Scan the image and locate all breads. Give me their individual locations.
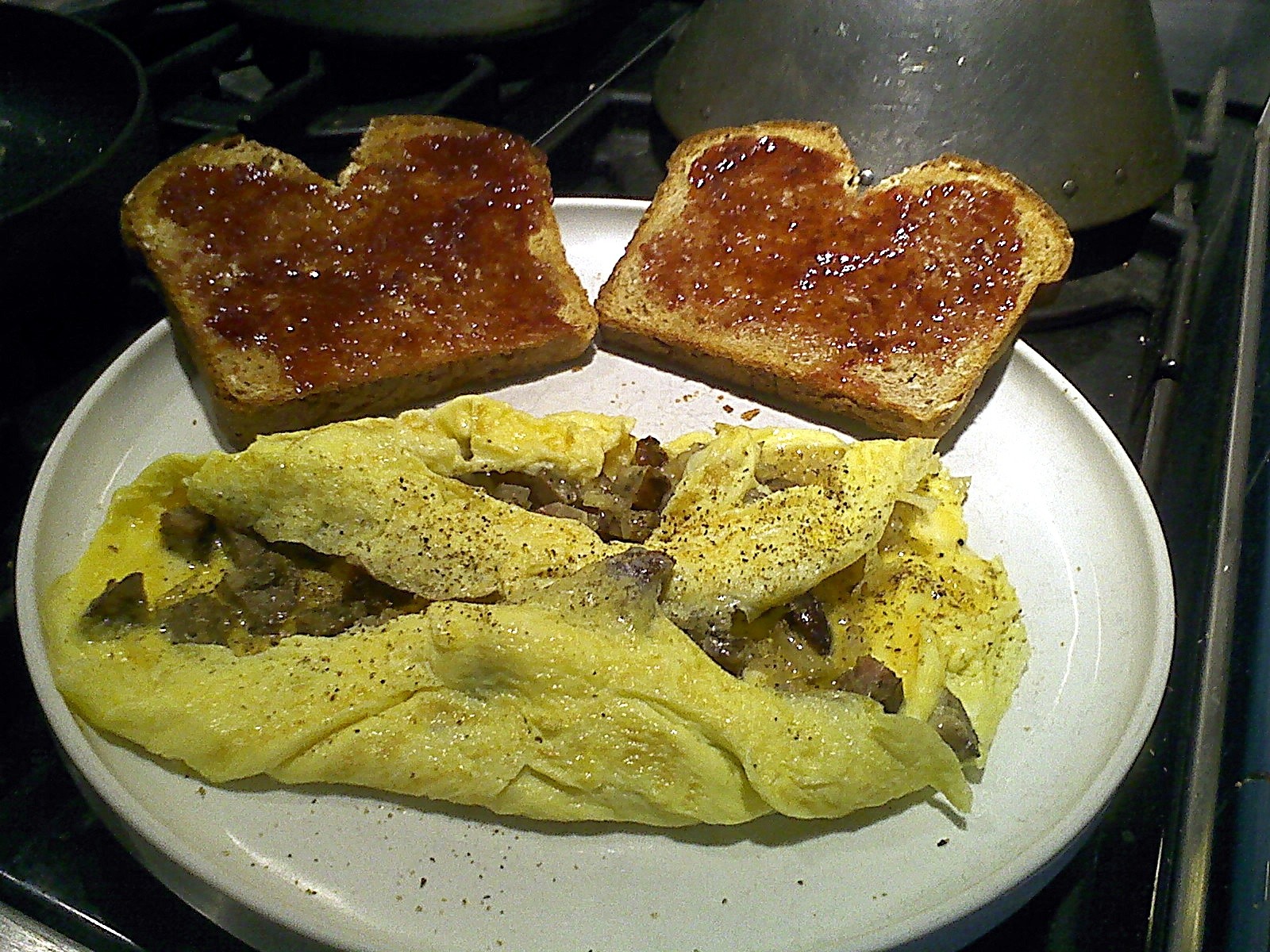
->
[121,115,598,454]
[593,120,1074,439]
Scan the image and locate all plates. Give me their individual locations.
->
[15,194,1179,952]
[1,1,149,219]
